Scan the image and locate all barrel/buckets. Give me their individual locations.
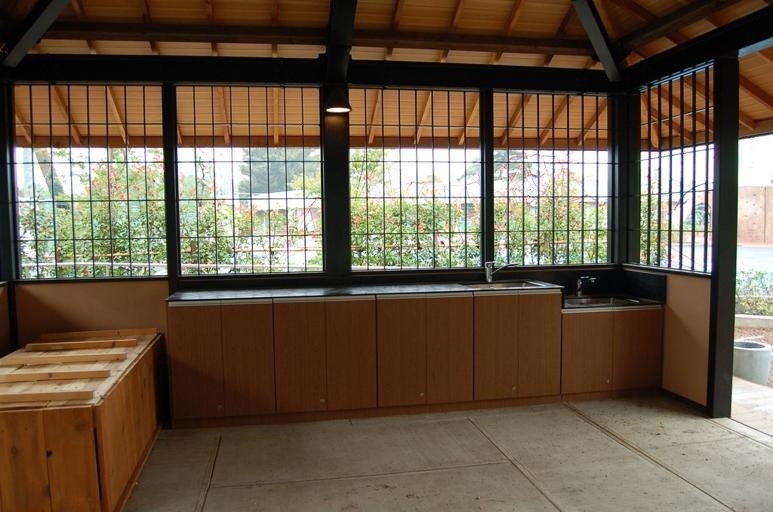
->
[732,338,773,384]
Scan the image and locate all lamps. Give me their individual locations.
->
[321,38,352,114]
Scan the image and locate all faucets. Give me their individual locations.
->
[492,262,520,277]
[576,274,597,298]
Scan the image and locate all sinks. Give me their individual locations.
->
[460,279,542,289]
[565,296,633,307]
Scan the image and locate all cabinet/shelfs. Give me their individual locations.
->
[0,332,162,510]
